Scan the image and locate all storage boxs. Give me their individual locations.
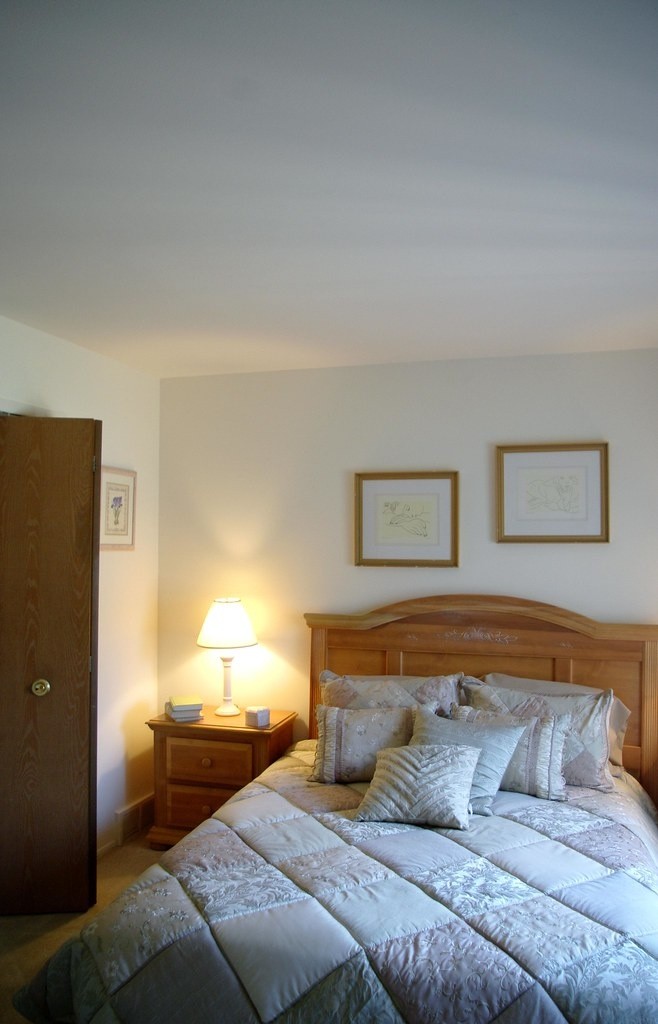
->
[244,706,270,727]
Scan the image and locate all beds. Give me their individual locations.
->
[14,594,658,1024]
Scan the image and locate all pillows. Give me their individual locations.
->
[306,669,633,831]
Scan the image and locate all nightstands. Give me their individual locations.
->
[144,705,298,850]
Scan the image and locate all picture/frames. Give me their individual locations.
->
[496,441,611,544]
[100,465,138,551]
[353,470,459,568]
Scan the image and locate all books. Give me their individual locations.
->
[165,695,205,722]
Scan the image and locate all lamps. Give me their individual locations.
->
[196,598,257,716]
